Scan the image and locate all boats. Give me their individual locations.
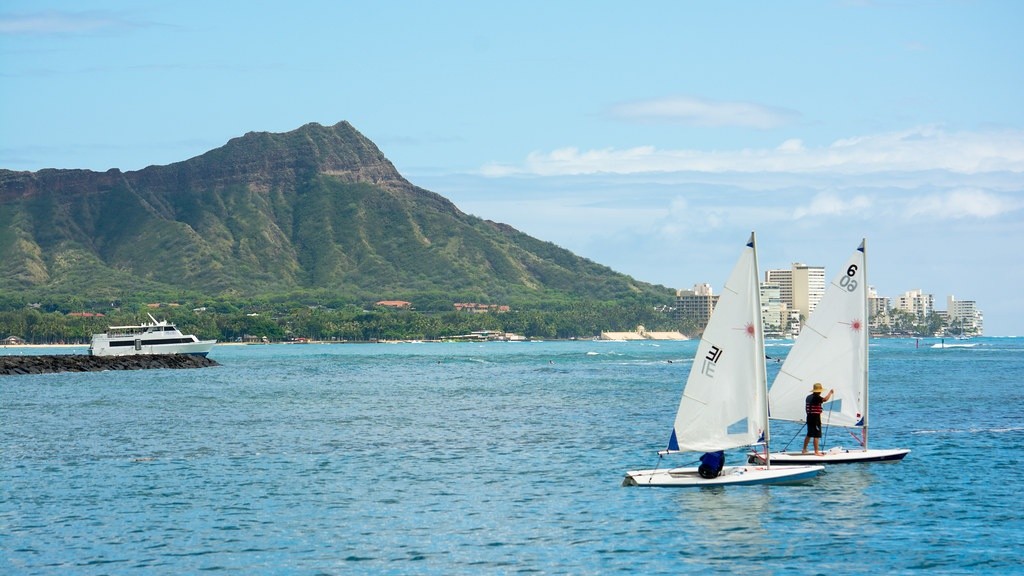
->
[87,311,217,357]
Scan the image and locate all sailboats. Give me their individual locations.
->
[744,237,912,464]
[622,232,826,489]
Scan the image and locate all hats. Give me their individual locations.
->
[809,383,824,392]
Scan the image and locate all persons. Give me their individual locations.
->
[802,384,834,456]
[698,450,725,478]
